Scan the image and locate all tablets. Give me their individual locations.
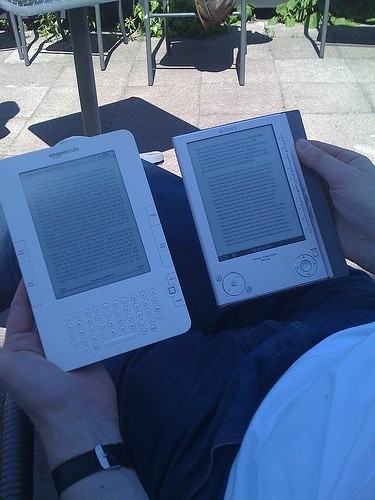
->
[173,112,333,309]
[0,129,191,374]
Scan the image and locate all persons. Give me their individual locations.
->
[0,134,375,500]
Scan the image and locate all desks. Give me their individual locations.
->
[1,0,118,139]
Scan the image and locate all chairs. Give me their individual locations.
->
[10,1,127,71]
[144,1,246,86]
[303,0,331,58]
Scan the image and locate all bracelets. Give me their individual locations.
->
[52,441,136,498]
